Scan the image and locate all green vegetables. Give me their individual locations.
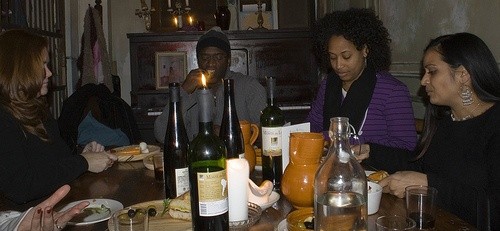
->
[160,199,170,216]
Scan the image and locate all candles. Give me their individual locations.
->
[185,0,189,6]
[167,0,171,8]
[224,157,250,225]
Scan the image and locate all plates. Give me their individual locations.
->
[277,218,288,231]
[108,199,194,231]
[363,169,377,176]
[108,145,160,161]
[59,198,124,225]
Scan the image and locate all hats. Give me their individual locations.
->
[195,25,231,55]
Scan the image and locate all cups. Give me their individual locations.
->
[113,207,149,231]
[405,185,439,231]
[375,215,416,231]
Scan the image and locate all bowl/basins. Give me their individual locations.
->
[348,180,382,215]
[288,209,336,231]
[229,202,261,231]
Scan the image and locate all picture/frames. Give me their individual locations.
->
[155,51,188,91]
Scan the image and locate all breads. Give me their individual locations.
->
[368,170,388,181]
[168,190,192,221]
[118,146,140,155]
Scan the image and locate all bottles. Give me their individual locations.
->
[279,132,329,208]
[164,82,189,199]
[189,87,229,231]
[239,120,259,172]
[219,79,243,159]
[217,6,231,31]
[313,117,369,231]
[260,75,285,189]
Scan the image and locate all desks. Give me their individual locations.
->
[17,158,477,231]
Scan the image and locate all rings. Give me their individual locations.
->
[56,224,64,229]
[108,159,112,165]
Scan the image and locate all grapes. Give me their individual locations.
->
[127,205,156,218]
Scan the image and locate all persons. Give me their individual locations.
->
[351,33,500,231]
[0,184,90,231]
[0,29,114,218]
[153,29,267,143]
[305,7,417,151]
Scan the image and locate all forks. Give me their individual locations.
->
[116,154,134,163]
